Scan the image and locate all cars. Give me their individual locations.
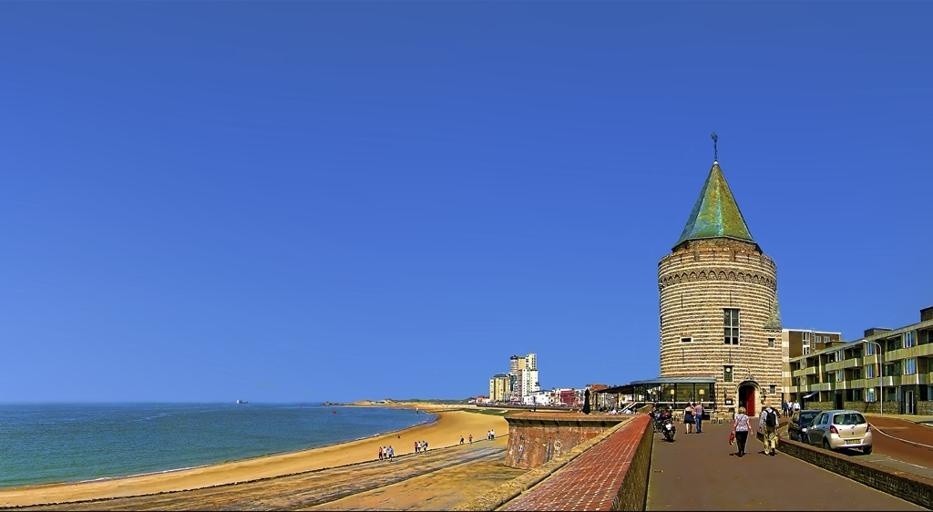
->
[787,409,872,455]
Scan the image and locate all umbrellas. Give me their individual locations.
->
[583,389,590,414]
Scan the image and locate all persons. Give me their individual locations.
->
[683,402,705,434]
[597,403,639,415]
[378,445,395,461]
[757,400,780,456]
[469,434,472,444]
[414,440,428,454]
[781,399,801,418]
[459,435,464,445]
[487,429,495,439]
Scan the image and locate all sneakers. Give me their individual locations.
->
[735,451,744,457]
[766,447,775,456]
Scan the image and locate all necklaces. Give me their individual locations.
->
[732,406,752,458]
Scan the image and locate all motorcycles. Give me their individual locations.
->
[648,409,676,442]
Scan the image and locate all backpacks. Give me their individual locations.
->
[764,409,776,426]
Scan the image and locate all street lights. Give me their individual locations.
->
[861,339,883,416]
